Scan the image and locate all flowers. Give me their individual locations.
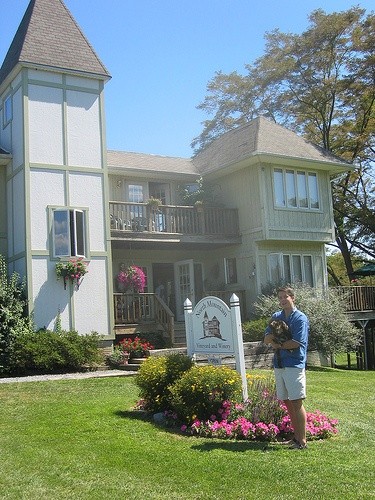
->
[55,256,88,285]
[114,263,147,293]
[118,336,154,352]
[120,350,131,361]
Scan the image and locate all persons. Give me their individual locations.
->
[263,287,308,449]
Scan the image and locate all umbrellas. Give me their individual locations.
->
[351,264,375,276]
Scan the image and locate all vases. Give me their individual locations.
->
[124,360,128,365]
[133,351,143,358]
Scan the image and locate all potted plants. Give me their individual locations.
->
[195,200,204,213]
[146,198,161,210]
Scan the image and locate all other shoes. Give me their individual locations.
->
[290,444,306,449]
[289,439,298,444]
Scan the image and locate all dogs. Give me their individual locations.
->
[269,320,293,369]
